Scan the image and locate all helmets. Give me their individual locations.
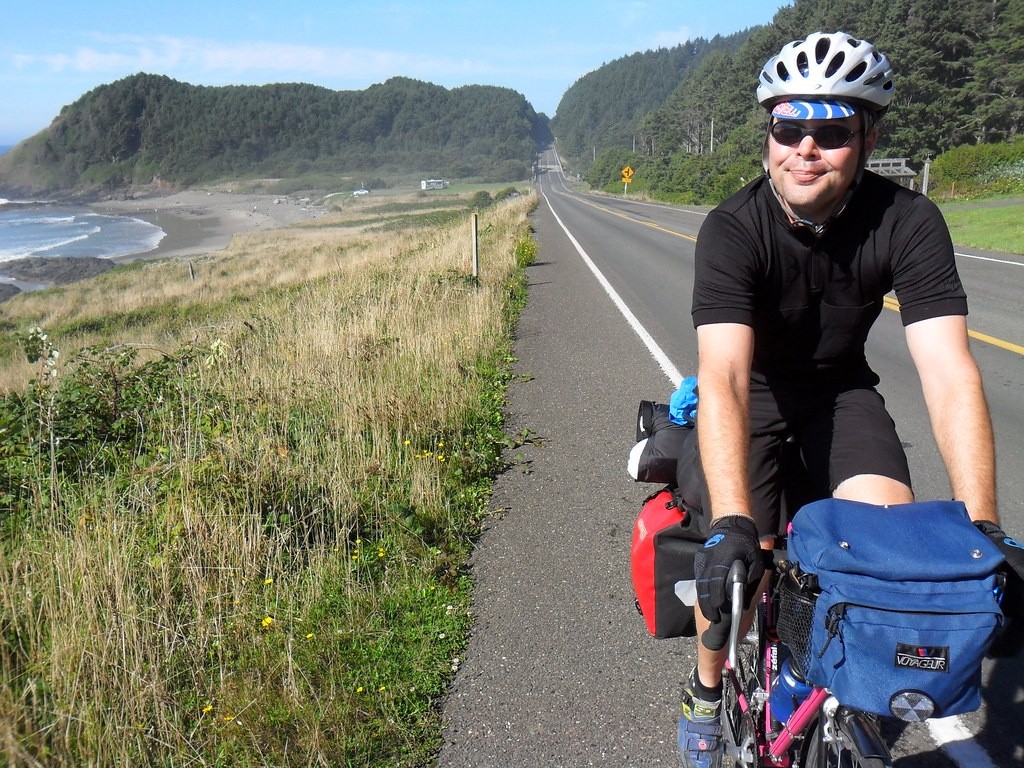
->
[757,29,899,122]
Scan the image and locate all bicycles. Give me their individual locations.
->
[720,432,1017,768]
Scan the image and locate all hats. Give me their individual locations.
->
[772,63,856,121]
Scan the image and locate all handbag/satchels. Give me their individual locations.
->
[629,489,697,638]
[777,498,1006,716]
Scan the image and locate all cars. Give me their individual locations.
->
[352,189,369,196]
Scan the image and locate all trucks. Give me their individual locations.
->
[421,180,450,190]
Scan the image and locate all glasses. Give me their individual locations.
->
[766,114,863,148]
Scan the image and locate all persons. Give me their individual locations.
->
[677,32,1024,768]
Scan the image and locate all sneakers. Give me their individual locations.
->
[677,664,728,768]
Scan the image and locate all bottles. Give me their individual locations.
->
[768,657,813,725]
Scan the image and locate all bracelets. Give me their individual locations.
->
[710,513,756,531]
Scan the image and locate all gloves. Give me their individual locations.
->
[971,520,1024,626]
[699,513,766,620]
